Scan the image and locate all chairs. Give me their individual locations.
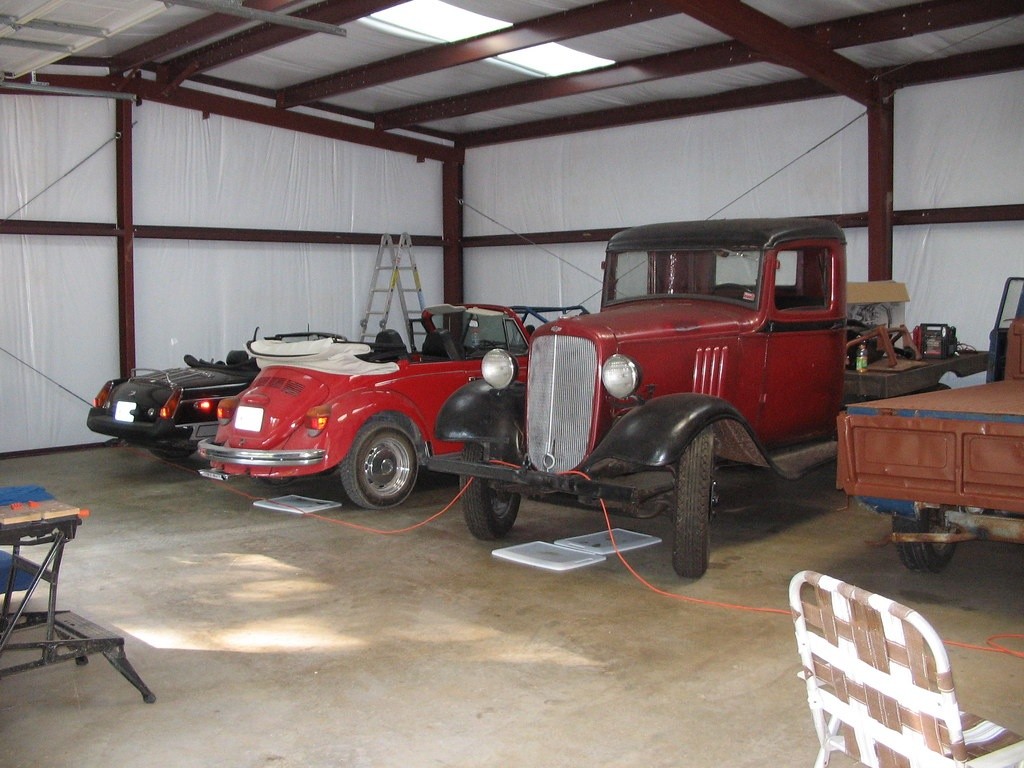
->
[424,329,467,361]
[375,329,409,364]
[789,570,1024,768]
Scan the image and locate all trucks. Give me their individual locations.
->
[425,215,988,582]
[834,275,1024,573]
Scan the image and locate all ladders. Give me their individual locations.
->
[358,232,427,354]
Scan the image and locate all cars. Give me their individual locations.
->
[86,329,347,461]
[198,304,536,511]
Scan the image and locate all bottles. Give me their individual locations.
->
[856,343,868,375]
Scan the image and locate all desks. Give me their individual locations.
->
[0,485,158,704]
